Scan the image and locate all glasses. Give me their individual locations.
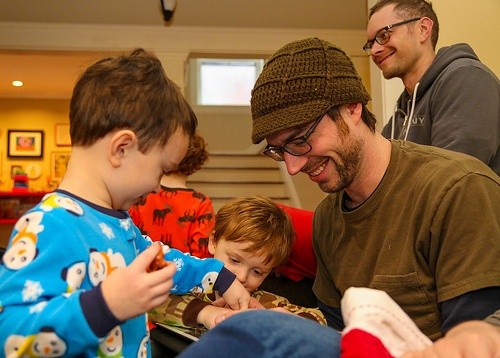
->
[362,17,421,56]
[262,112,325,161]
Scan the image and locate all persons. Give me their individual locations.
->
[363,0,500,177]
[147,36,500,358]
[0,48,251,358]
[127,128,217,330]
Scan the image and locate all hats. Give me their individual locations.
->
[251,36,372,144]
